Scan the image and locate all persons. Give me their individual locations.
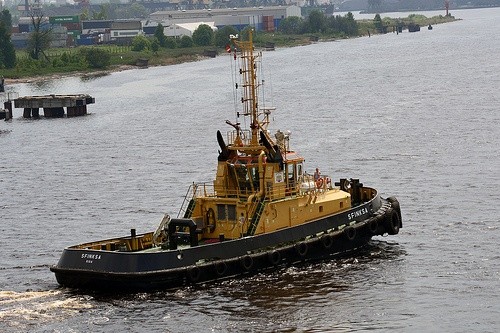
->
[303,170,311,183]
[313,168,322,188]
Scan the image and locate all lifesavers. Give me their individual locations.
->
[317,178,324,188]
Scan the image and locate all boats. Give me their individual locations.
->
[50,27,403,294]
